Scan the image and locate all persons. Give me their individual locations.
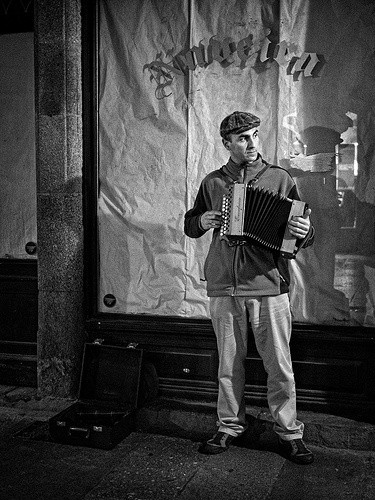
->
[182,112,314,459]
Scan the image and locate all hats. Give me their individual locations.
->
[220,111,260,139]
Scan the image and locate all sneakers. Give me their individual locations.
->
[278,434,314,465]
[198,431,235,454]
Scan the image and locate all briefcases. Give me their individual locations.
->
[47,339,146,450]
